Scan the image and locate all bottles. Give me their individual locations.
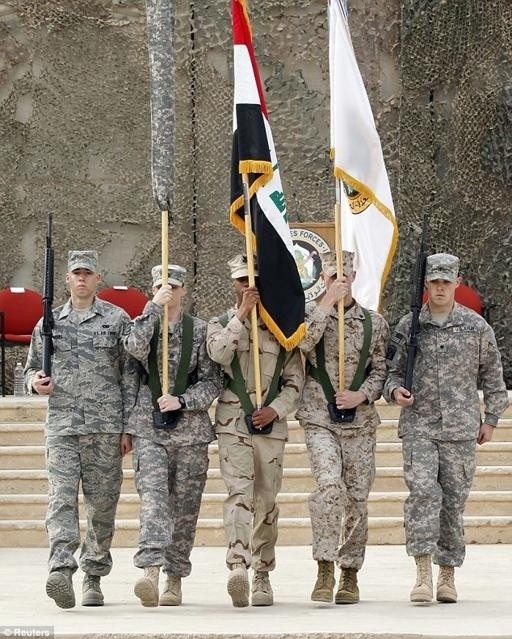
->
[13,362,26,397]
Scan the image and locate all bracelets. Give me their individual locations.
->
[178,395,185,408]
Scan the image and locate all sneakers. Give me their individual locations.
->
[46,567,75,608]
[81,574,104,606]
[311,560,335,602]
[252,570,273,606]
[135,566,160,607]
[411,554,433,601]
[160,576,182,605]
[227,563,249,607]
[436,566,457,602]
[335,568,359,603]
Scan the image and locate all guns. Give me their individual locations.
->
[404,213,427,399]
[41,214,54,386]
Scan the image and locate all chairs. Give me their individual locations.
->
[96,284,148,320]
[0,287,47,344]
[422,284,498,328]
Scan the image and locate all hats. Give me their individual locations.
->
[425,254,460,282]
[320,251,355,277]
[151,265,187,287]
[227,254,260,278]
[68,251,98,273]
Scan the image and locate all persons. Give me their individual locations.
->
[206,253,304,607]
[295,250,388,608]
[24,248,136,610]
[124,264,223,608]
[383,253,510,605]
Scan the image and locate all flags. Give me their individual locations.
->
[227,3,307,351]
[326,2,398,312]
[146,0,175,210]
